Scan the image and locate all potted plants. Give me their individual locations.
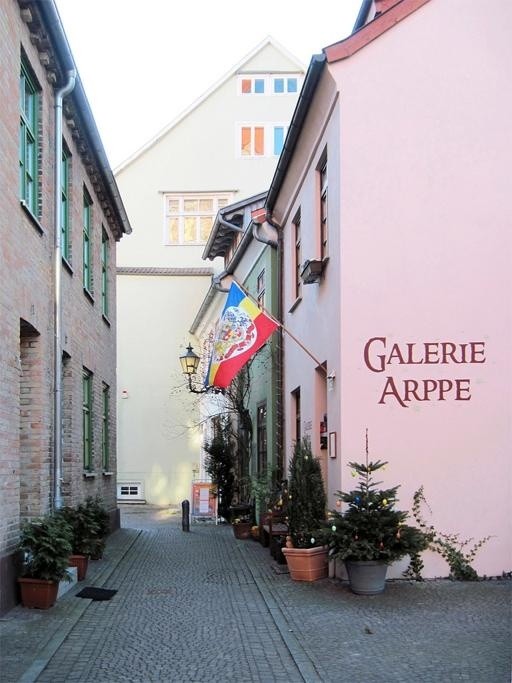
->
[279,435,435,597]
[13,496,112,609]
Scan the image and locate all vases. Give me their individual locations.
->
[234,518,252,538]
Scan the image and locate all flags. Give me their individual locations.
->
[204,279,280,389]
